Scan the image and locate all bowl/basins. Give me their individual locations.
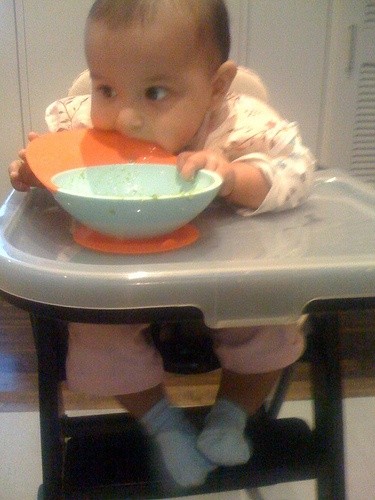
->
[25,128,223,254]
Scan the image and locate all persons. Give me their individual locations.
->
[10,0,316,486]
